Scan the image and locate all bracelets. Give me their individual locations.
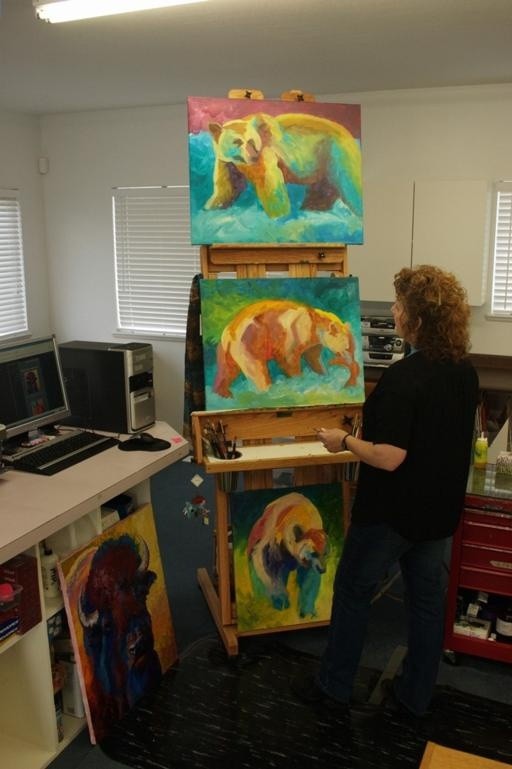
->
[342,433,353,450]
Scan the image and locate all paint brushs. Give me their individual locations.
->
[201,418,239,459]
[340,413,362,482]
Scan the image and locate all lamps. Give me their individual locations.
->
[35,2,192,25]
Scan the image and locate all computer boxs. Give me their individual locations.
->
[55,340,156,435]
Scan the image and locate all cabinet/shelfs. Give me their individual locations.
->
[444,419,511,663]
[191,89,361,656]
[363,180,492,306]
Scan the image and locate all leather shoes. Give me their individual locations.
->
[290,675,351,715]
[380,678,432,724]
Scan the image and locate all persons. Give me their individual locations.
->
[286,263,480,719]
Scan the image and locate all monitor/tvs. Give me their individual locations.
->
[0,334,72,464]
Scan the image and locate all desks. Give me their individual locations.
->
[0,420,192,769]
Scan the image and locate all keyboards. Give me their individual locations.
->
[13,430,121,477]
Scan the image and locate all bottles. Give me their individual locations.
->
[42,550,59,598]
[474,437,488,463]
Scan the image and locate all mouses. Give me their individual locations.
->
[127,433,154,445]
